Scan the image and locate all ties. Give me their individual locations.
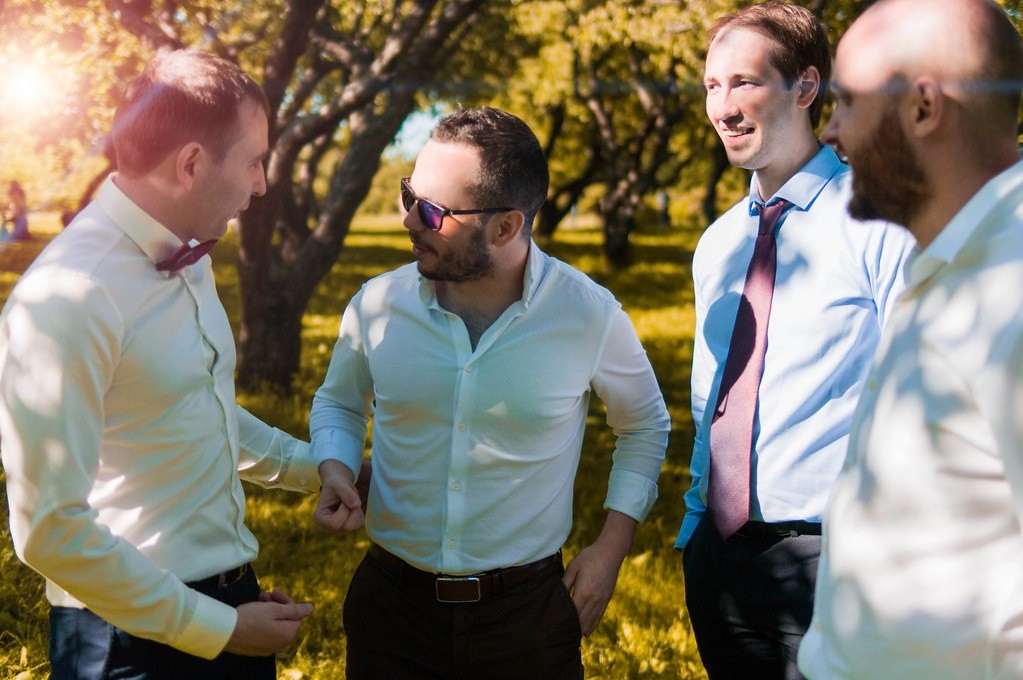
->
[707,199,792,539]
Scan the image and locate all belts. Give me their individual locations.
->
[703,514,821,537]
[368,542,559,603]
[183,561,249,592]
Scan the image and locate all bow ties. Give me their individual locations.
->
[154,238,218,273]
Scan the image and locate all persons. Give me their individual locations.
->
[0,46,371,680]
[799,0,1023,679]
[308,108,671,680]
[672,0,914,680]
[0,180,29,241]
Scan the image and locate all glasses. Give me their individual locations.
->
[400,176,520,233]
